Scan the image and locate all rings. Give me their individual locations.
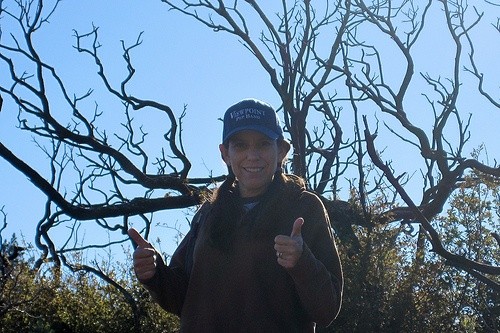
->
[276,251,284,256]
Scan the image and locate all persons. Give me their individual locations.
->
[127,98,338,333]
